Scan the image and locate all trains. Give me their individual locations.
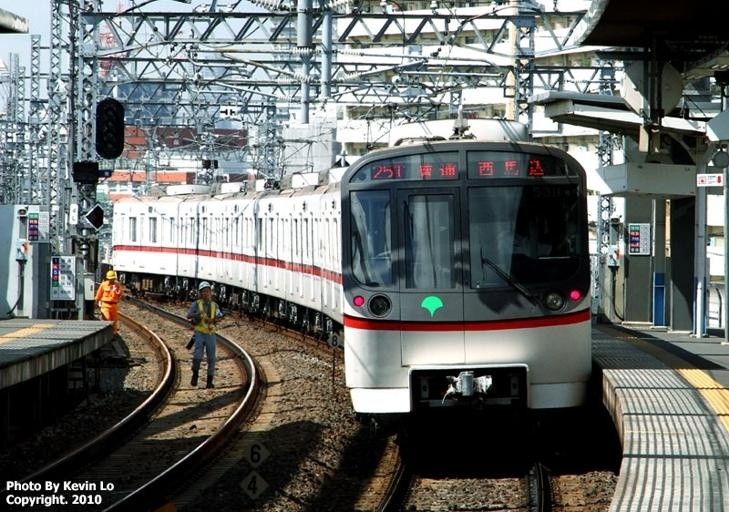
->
[110,138,593,455]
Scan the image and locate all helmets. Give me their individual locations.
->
[106,270,117,279]
[198,281,211,291]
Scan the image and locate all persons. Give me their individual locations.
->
[94,270,123,341]
[186,280,223,388]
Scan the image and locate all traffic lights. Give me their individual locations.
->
[97,97,125,159]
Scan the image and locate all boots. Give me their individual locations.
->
[207,375,214,388]
[191,371,198,386]
